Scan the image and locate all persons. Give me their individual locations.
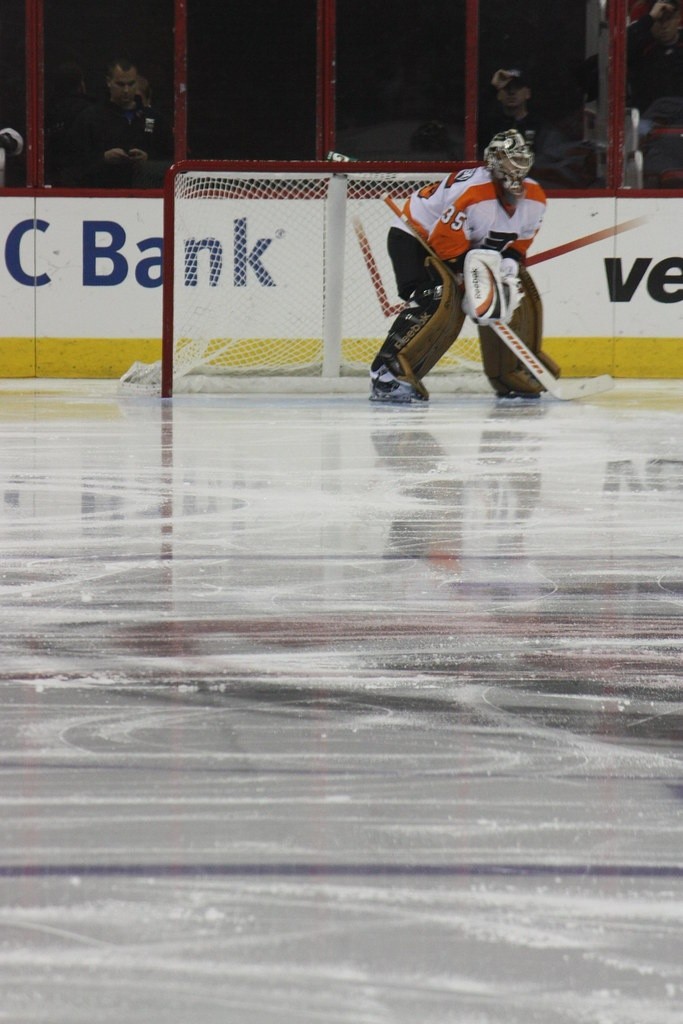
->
[369,128,562,408]
[53,57,174,188]
[627,0,683,189]
[476,68,563,180]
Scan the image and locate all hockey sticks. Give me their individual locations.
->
[379,190,616,400]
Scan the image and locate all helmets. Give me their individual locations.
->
[483,129,535,178]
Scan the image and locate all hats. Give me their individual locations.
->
[503,63,531,86]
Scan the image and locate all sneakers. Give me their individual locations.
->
[494,390,541,409]
[369,364,420,404]
